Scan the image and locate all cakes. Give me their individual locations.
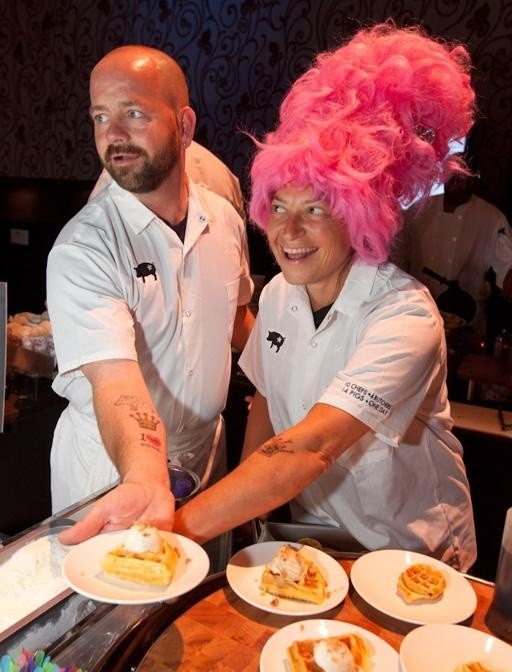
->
[455,662,487,672]
[259,543,326,605]
[286,632,375,672]
[101,517,180,588]
[397,562,447,604]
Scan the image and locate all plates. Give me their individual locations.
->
[225,540,350,617]
[61,530,211,606]
[259,619,401,672]
[400,625,512,672]
[351,549,478,625]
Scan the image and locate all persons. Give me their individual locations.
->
[83,134,250,233]
[42,44,257,606]
[388,152,512,298]
[166,25,478,580]
